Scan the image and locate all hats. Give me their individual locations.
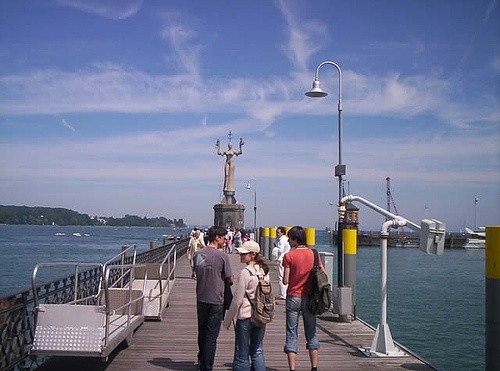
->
[234,241,261,254]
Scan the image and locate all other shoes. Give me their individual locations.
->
[192,273,197,280]
[275,296,286,300]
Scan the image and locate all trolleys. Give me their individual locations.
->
[29,261,146,367]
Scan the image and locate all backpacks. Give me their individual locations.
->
[245,267,276,327]
[307,249,331,315]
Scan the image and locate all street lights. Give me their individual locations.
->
[245,177,258,242]
[303,60,346,287]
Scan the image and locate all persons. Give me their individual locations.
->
[185,225,250,279]
[216,137,245,191]
[192,226,234,371]
[282,226,326,371]
[223,242,270,371]
[272,227,291,300]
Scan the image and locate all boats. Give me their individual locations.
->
[333,176,469,247]
[457,196,487,248]
